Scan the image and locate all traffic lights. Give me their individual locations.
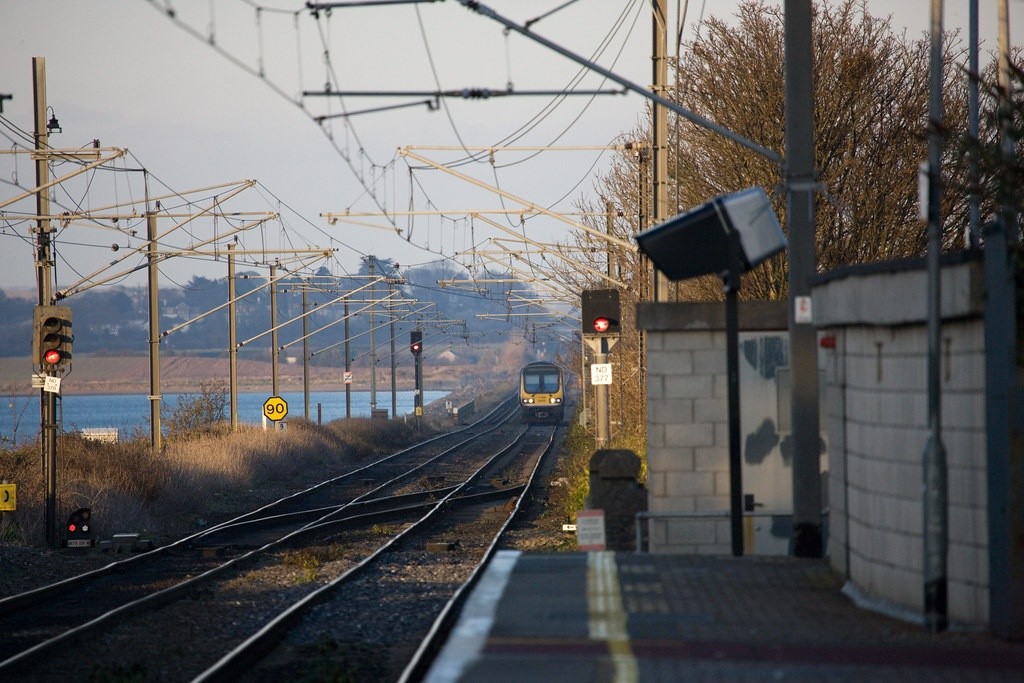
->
[410,331,423,354]
[39,313,63,366]
[581,288,621,338]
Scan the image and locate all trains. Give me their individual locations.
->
[518,360,566,424]
